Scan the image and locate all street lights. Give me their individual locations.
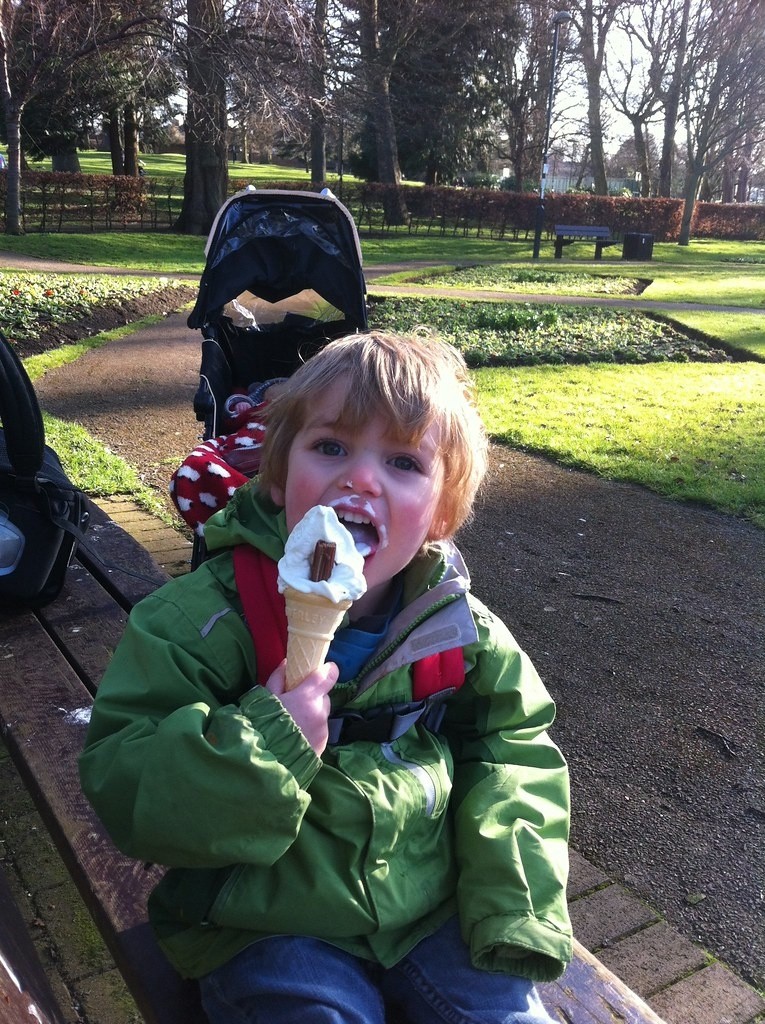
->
[531,10,573,257]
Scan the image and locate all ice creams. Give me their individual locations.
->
[276,503,366,694]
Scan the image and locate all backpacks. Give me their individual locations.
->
[0,329,91,608]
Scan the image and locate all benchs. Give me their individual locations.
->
[553,224,619,260]
[1,495,666,1024]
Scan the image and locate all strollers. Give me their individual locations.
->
[188,187,372,578]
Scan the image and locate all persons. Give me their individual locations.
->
[78,329,572,1024]
[224,376,287,415]
